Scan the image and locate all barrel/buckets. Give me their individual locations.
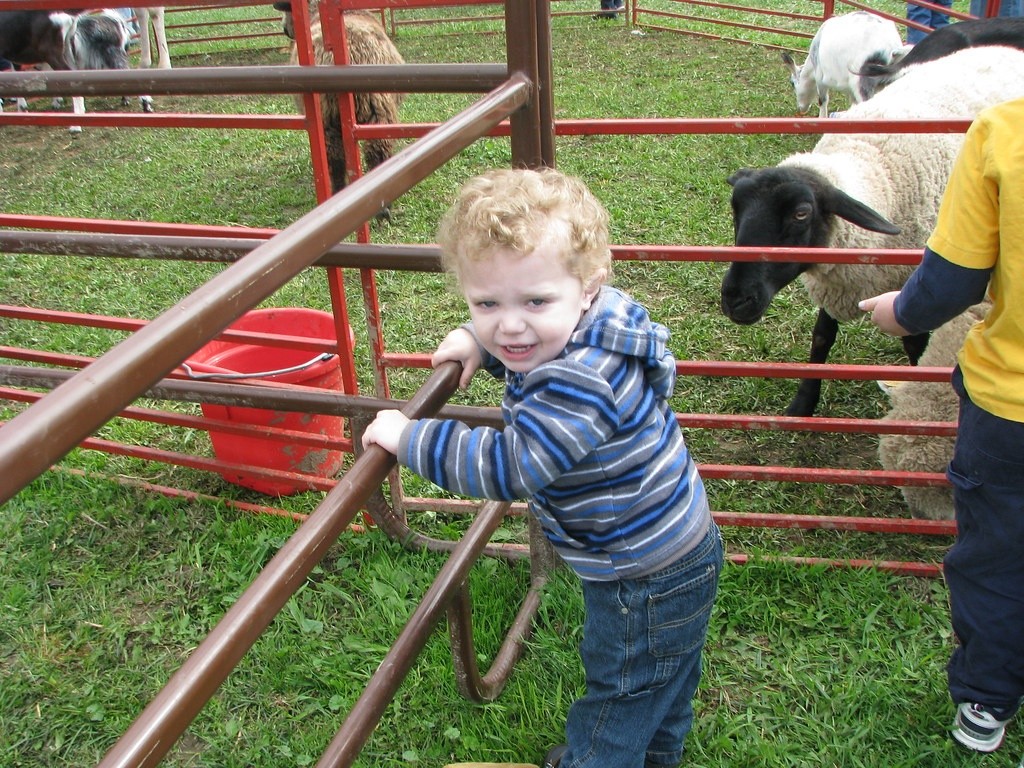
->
[178,307,357,497]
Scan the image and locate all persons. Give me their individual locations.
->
[361,168,725,768]
[859,97,1024,751]
[907,0,953,44]
[592,0,625,20]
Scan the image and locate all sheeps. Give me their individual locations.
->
[718,7,1024,548]
[1,0,174,134]
[272,0,409,229]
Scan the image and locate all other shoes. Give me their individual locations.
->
[592,11,618,20]
[616,5,625,10]
[952,694,1024,753]
